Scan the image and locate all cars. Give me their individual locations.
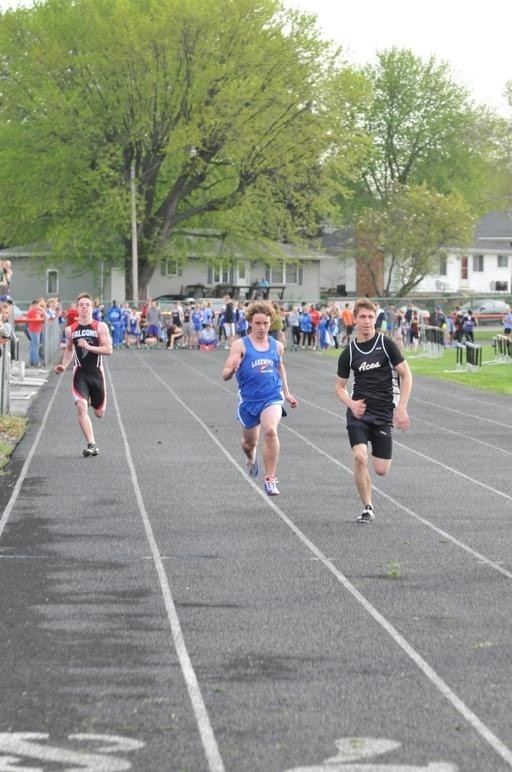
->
[381,302,430,323]
[454,298,510,327]
[14,305,29,331]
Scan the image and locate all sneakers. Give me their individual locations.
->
[249,458,259,477]
[83,444,99,457]
[356,506,376,523]
[264,477,280,496]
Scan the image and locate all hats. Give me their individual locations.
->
[0,295,12,305]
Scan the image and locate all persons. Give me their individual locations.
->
[374,303,477,354]
[221,298,298,496]
[92,297,250,351]
[333,297,414,524]
[27,297,78,367]
[54,291,115,458]
[0,259,18,356]
[268,300,354,350]
[502,310,511,335]
